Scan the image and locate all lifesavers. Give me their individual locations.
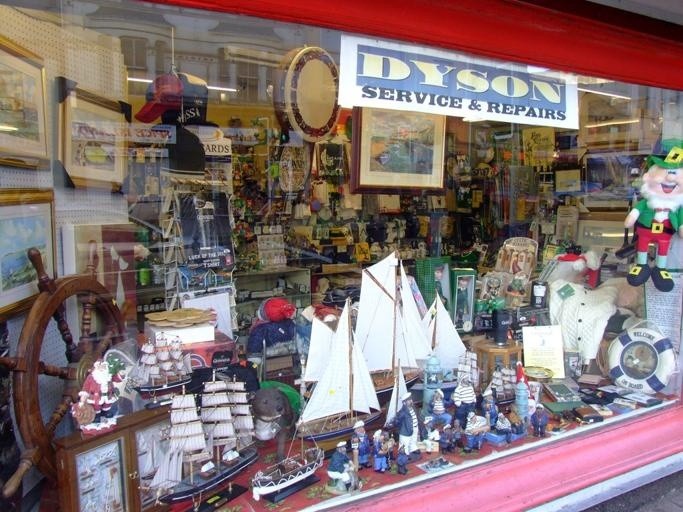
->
[607,327,675,395]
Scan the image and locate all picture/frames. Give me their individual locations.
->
[0,33,54,173]
[552,168,584,194]
[348,102,449,198]
[0,185,59,320]
[49,75,132,193]
[577,144,651,214]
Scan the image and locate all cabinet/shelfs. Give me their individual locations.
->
[76,265,310,342]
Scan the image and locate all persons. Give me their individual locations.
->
[434,265,525,328]
[328,373,548,491]
[247,297,296,354]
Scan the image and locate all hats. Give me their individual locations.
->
[131,73,185,125]
[144,120,209,178]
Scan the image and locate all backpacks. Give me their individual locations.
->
[160,68,213,129]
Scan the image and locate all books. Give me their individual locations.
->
[543,352,663,423]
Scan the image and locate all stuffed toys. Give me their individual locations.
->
[624,139,683,292]
